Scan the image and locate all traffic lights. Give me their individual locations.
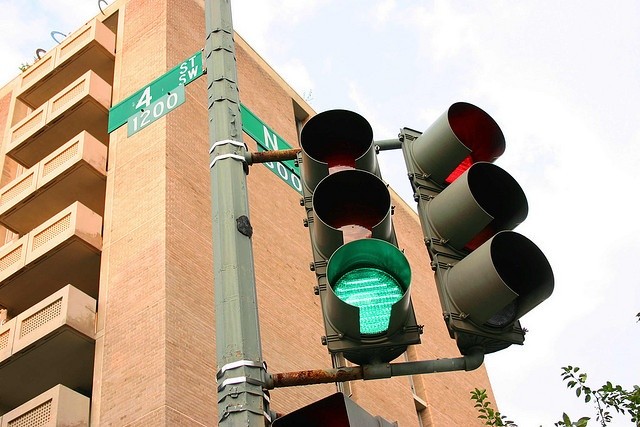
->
[294,108,425,365]
[397,98,554,357]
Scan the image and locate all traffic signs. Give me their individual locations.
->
[108,49,205,138]
[238,102,305,196]
